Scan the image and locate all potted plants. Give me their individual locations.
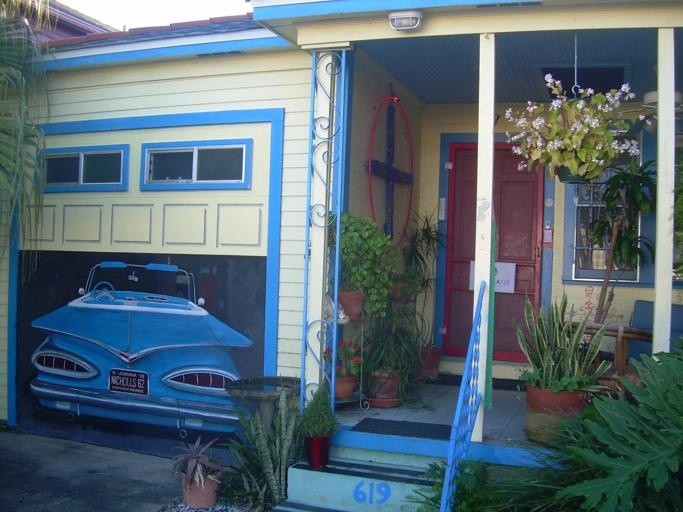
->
[303,376,336,471]
[173,435,220,509]
[392,209,441,300]
[340,212,398,321]
[510,289,614,446]
[366,333,412,408]
[416,320,442,376]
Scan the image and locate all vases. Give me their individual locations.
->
[556,159,598,186]
[330,371,356,401]
[335,377,357,400]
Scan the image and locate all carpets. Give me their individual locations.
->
[349,418,452,441]
[426,373,530,392]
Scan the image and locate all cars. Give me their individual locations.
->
[21,259,259,438]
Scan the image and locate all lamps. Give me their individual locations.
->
[390,9,424,33]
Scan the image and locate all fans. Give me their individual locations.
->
[619,63,682,123]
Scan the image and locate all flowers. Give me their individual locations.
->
[504,73,639,170]
[324,336,366,378]
